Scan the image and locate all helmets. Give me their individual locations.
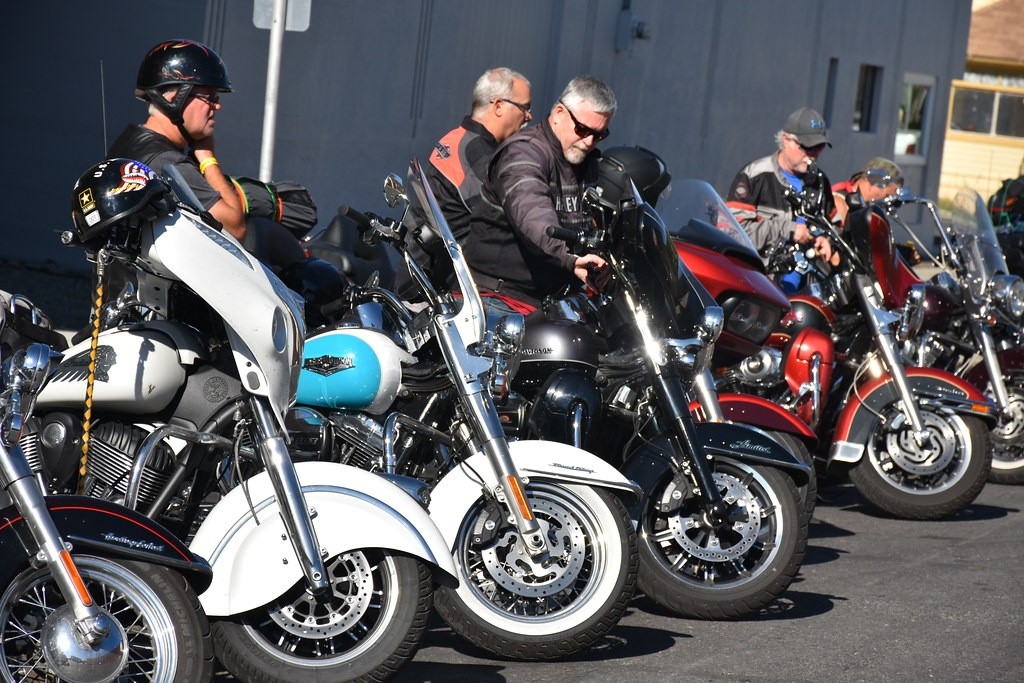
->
[135,39,233,124]
[70,158,172,243]
[598,146,670,207]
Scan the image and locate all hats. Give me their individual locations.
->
[783,106,833,149]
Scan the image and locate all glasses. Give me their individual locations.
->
[491,98,531,113]
[188,94,219,103]
[793,139,814,151]
[559,99,610,141]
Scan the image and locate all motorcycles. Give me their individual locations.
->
[711,186,1024,522]
[0,290,217,683]
[29,160,460,683]
[285,157,646,663]
[492,173,815,620]
[653,178,818,522]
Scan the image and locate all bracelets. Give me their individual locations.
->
[199,157,218,175]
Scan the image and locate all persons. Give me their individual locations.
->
[463,74,618,331]
[831,158,921,268]
[717,107,842,293]
[108,37,246,337]
[399,66,532,292]
[986,156,1024,280]
